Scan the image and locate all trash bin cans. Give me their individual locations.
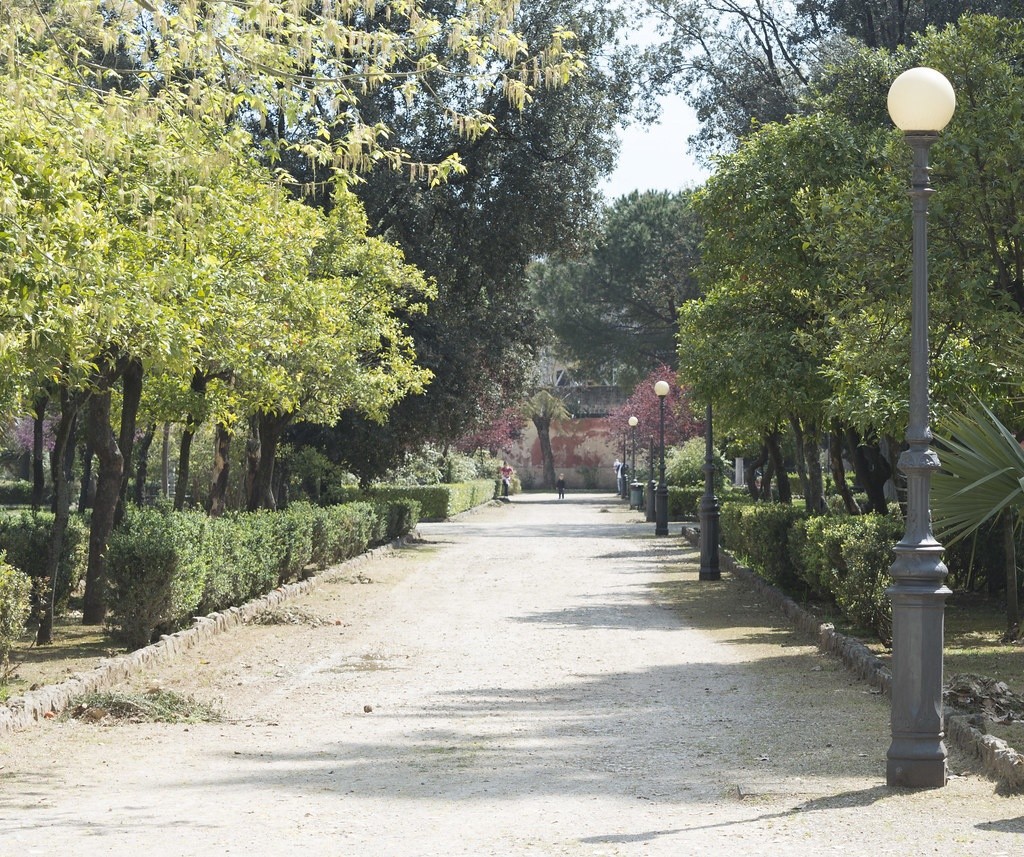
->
[629,482,645,510]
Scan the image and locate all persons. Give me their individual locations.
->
[555,474,565,499]
[615,458,628,496]
[499,461,513,501]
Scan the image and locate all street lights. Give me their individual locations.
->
[629,417,639,483]
[888,67,957,791]
[655,380,670,536]
